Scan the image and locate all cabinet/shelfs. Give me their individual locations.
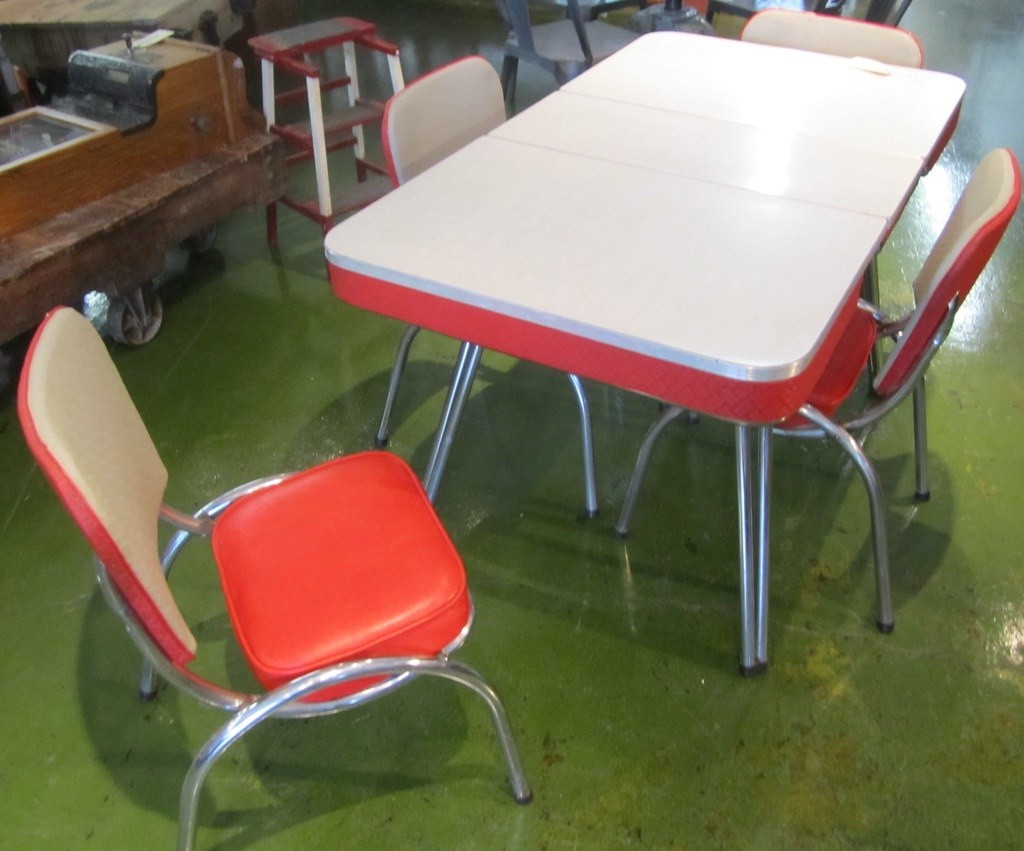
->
[247,13,404,243]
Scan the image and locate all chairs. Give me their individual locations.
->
[14,307,535,851]
[493,0,923,120]
[616,151,1024,635]
[376,56,604,517]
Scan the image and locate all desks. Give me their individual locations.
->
[320,28,965,677]
